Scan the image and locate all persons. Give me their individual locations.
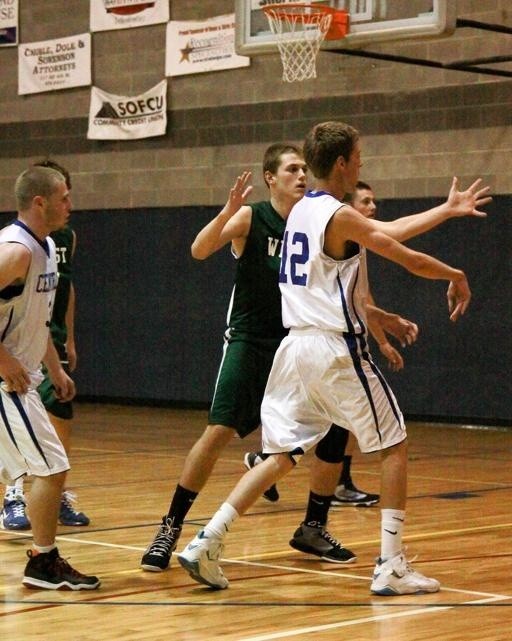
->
[241,178,381,507]
[0,163,103,593]
[138,143,494,571]
[0,159,89,528]
[171,115,473,594]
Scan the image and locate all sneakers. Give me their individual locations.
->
[330,481,380,506]
[290,521,357,564]
[0,488,101,591]
[244,452,279,501]
[370,558,440,596]
[140,516,229,589]
